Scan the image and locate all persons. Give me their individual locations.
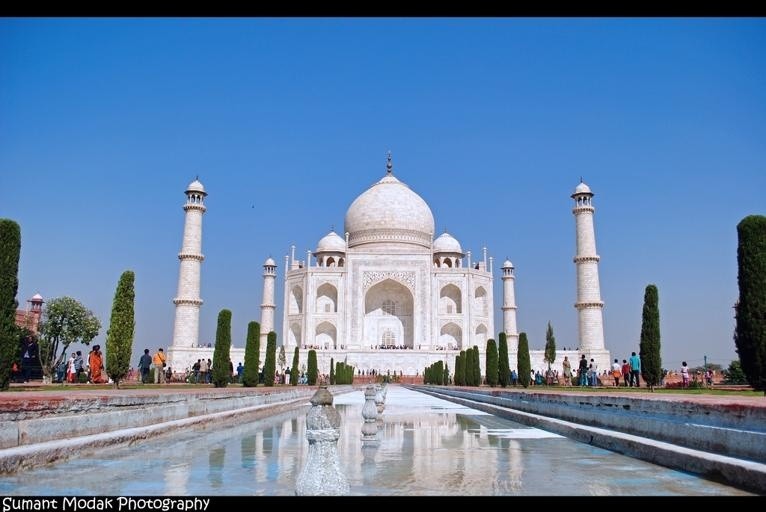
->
[20,336,37,383]
[43,363,52,384]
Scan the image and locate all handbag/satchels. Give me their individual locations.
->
[163,363,166,367]
[79,368,84,372]
